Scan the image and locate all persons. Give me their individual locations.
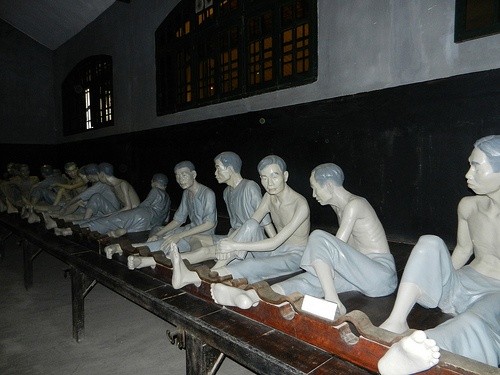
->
[170,156,310,290]
[209,162,398,319]
[127,151,277,270]
[104,161,218,259]
[54,173,171,237]
[378,133,500,375]
[0,152,140,229]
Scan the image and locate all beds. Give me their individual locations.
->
[1,206,500,375]
